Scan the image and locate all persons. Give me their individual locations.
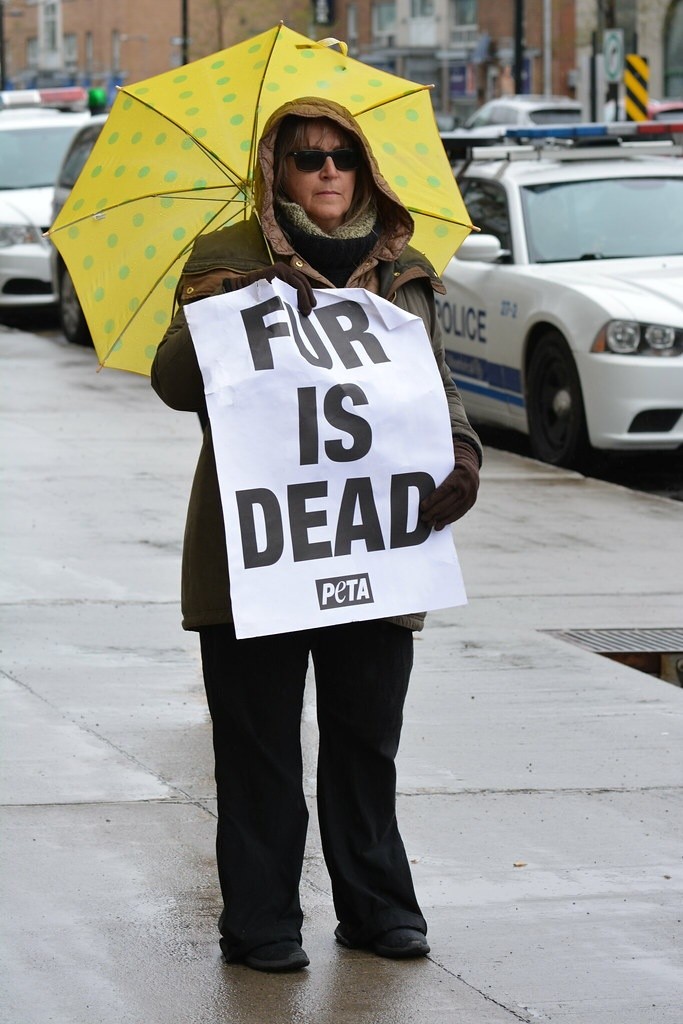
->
[149,95,487,975]
[493,64,515,97]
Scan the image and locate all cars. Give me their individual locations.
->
[425,117,683,473]
[647,98,683,145]
[0,81,110,349]
[464,94,587,147]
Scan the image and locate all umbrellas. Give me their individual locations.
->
[43,19,483,377]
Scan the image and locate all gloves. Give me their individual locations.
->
[223,261,317,317]
[419,441,480,531]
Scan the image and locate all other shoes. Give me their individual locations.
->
[219,937,310,969]
[334,923,430,957]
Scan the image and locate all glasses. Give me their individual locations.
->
[286,149,362,172]
